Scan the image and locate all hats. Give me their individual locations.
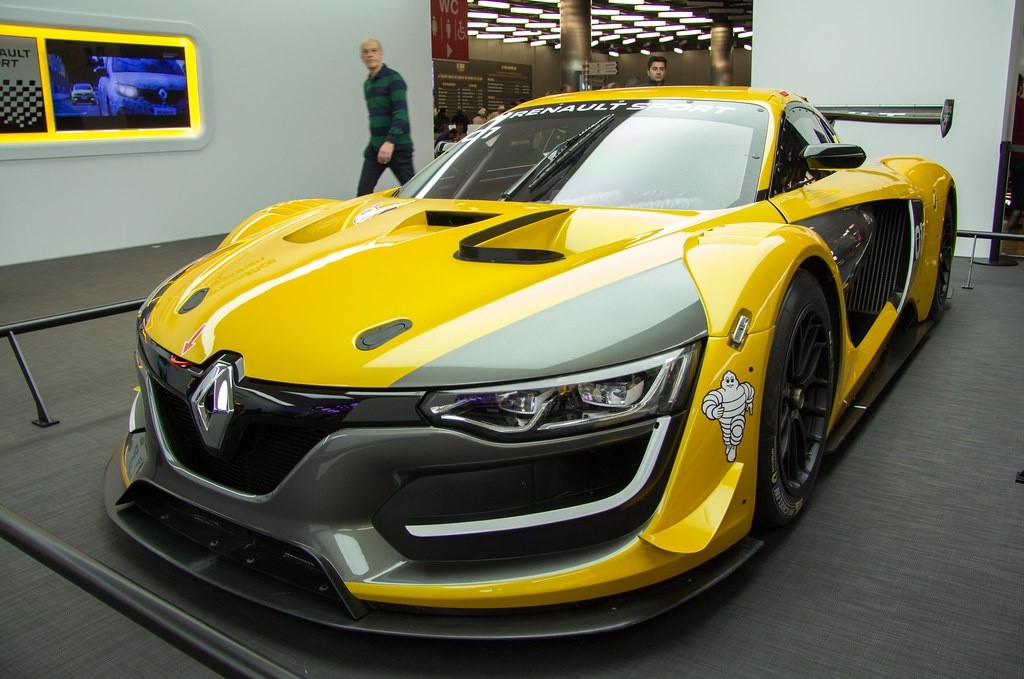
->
[477,108,487,117]
[499,105,505,110]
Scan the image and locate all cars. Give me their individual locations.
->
[71,82,96,105]
[94,58,186,118]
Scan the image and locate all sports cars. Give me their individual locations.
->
[98,84,957,640]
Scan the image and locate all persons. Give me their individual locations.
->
[434,100,526,159]
[608,83,619,88]
[641,56,667,86]
[357,38,415,197]
[539,85,571,151]
